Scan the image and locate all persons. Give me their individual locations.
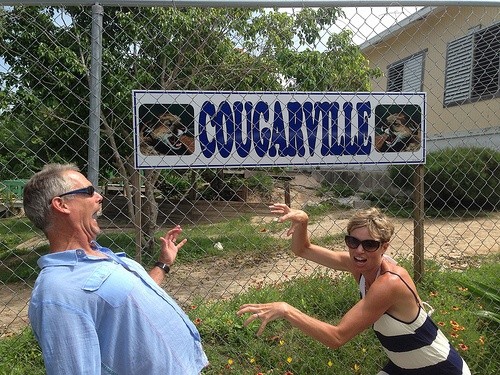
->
[22,164,210,375]
[235,201,476,375]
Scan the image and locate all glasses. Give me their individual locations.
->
[345,234,387,252]
[48,185,97,206]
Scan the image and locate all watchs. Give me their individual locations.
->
[154,260,169,275]
